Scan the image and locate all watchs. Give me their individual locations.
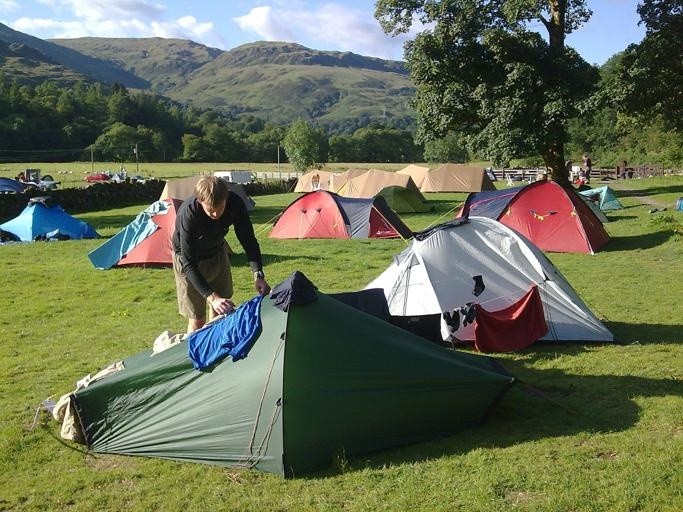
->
[255,270,266,278]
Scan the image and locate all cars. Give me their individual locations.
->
[84,174,110,183]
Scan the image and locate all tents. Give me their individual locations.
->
[53,271,518,482]
[360,215,614,346]
[0,162,625,269]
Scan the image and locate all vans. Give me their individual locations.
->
[214,171,260,184]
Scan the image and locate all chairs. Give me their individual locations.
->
[506,174,514,187]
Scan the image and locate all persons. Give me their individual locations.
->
[582,155,592,184]
[172,174,272,335]
